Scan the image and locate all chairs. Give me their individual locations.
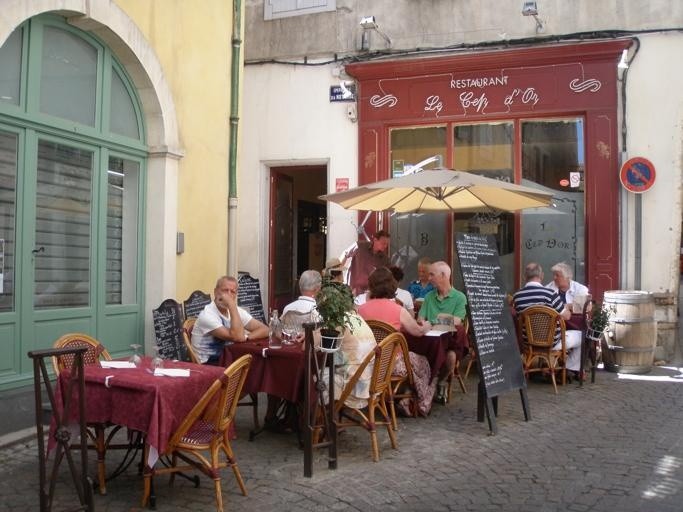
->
[51,333,132,495]
[280,310,322,332]
[317,333,403,462]
[137,354,251,512]
[447,314,466,404]
[183,316,202,367]
[518,305,566,396]
[365,318,417,430]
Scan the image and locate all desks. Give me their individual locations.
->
[400,316,465,341]
[62,353,226,472]
[227,327,327,435]
[509,307,587,324]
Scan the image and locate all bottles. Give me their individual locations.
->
[268,310,281,348]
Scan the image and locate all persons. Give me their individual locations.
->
[190,276,269,365]
[279,270,322,339]
[353,267,415,320]
[307,282,377,446]
[416,261,467,417]
[544,262,592,381]
[408,258,436,299]
[341,230,390,296]
[510,263,584,382]
[358,266,431,418]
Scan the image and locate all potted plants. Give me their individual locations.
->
[320,281,350,349]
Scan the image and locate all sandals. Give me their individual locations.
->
[396,399,413,417]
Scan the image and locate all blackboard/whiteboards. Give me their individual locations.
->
[184,289,210,319]
[152,298,190,362]
[454,230,526,400]
[236,271,268,326]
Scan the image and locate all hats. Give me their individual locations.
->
[321,257,343,278]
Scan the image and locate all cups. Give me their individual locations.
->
[151,346,164,373]
[283,315,297,345]
[128,343,142,369]
